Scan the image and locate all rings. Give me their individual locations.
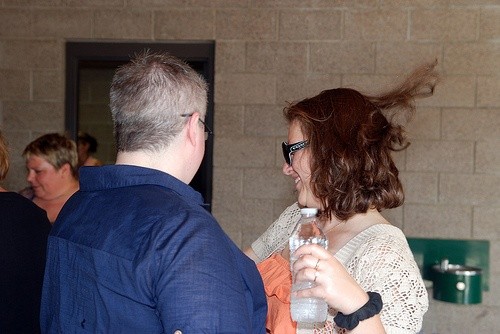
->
[313,269,320,281]
[314,258,321,270]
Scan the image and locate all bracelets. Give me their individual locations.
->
[333,291,383,331]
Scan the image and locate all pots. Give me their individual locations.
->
[433,263,483,304]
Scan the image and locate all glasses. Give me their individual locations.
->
[281,140,311,165]
[182,112,212,140]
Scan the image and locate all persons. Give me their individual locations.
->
[21,131,81,224]
[240,59,442,334]
[0,137,52,334]
[40,49,269,334]
[72,134,102,168]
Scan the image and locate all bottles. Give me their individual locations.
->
[289,207,328,323]
